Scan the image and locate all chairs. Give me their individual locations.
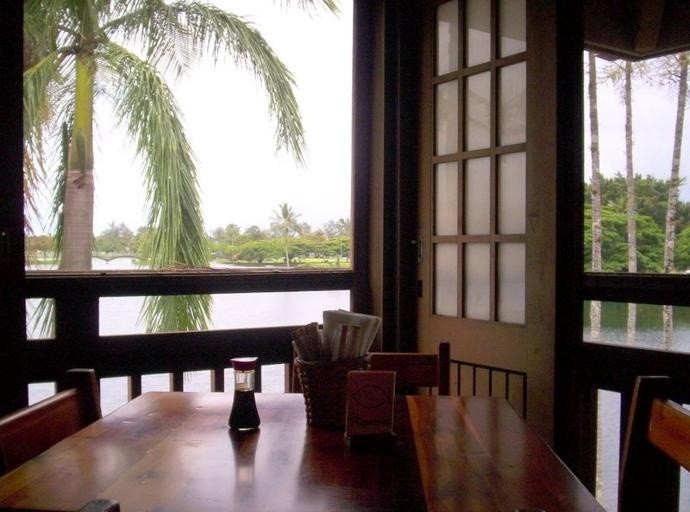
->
[0,366,101,478]
[288,340,451,396]
[615,376,690,512]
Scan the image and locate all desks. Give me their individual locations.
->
[0,392,609,512]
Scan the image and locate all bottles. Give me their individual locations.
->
[229,356,260,430]
